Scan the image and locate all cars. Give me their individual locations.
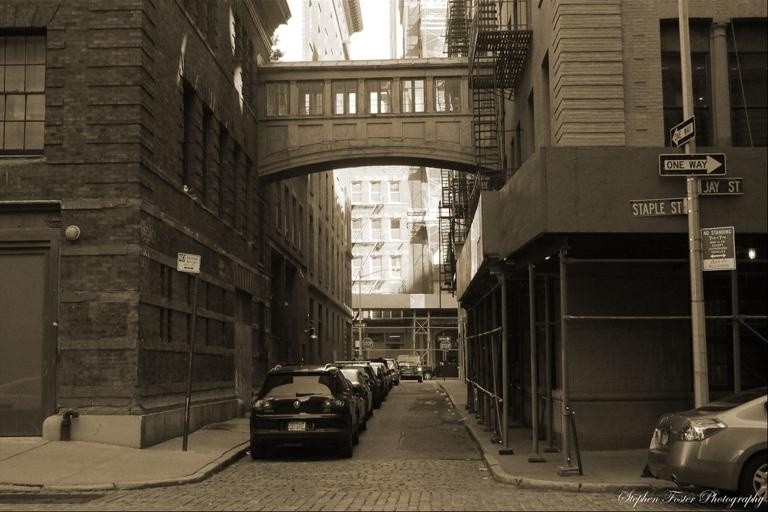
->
[645,386,768,510]
[251,355,458,460]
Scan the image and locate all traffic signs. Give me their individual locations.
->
[175,252,201,274]
[658,153,729,178]
[670,115,696,149]
[697,178,745,196]
[630,198,687,219]
